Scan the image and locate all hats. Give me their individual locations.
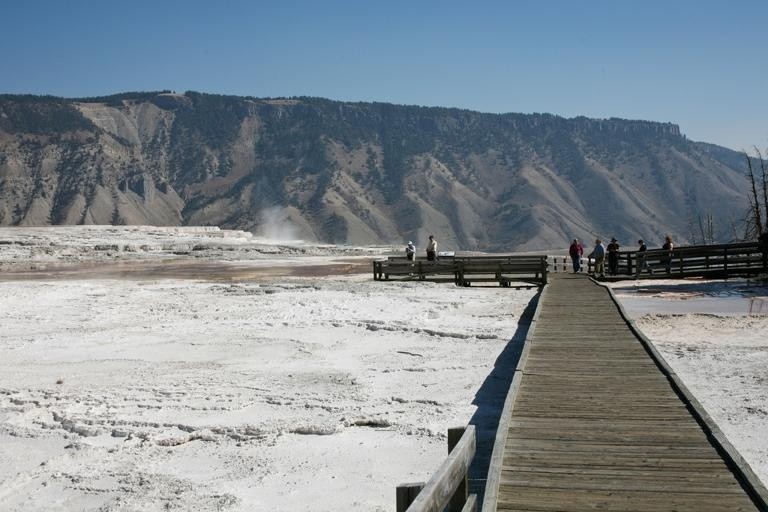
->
[611,238,618,242]
[408,240,412,244]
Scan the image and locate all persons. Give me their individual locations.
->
[426,235,437,266]
[569,238,584,274]
[606,237,620,276]
[588,239,605,278]
[660,235,674,274]
[633,239,654,277]
[406,240,417,276]
